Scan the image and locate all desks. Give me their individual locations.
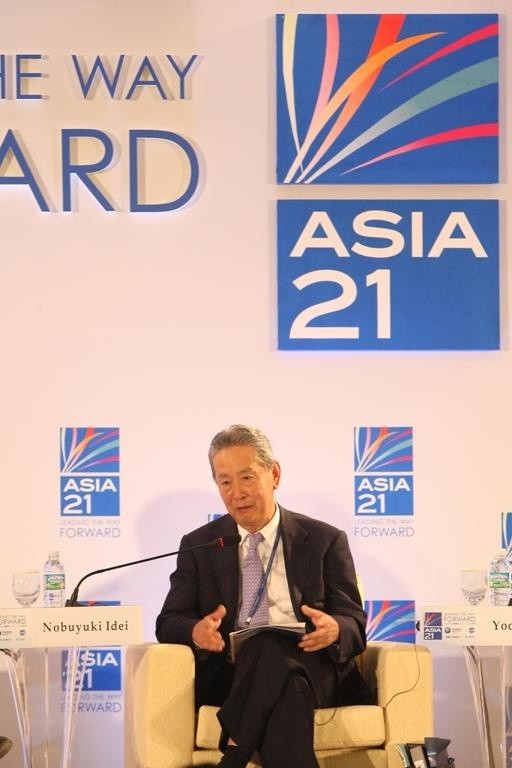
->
[415,619,512,768]
[1,606,144,768]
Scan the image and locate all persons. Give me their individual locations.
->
[153,421,370,767]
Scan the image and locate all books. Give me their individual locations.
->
[227,620,307,663]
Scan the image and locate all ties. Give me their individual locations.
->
[236,532,270,631]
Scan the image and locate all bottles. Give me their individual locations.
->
[488,545,512,605]
[43,550,67,608]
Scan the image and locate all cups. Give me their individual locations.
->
[12,569,42,609]
[460,565,488,604]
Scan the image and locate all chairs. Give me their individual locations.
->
[122,640,435,768]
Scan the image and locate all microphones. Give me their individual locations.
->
[64,533,241,607]
